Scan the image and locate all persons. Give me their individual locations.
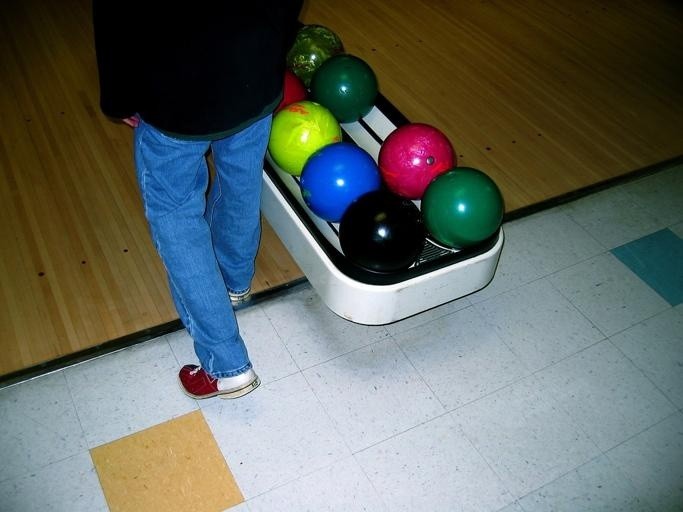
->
[94,0,303,400]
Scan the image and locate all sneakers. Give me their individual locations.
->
[177,364,262,400]
[226,286,252,307]
